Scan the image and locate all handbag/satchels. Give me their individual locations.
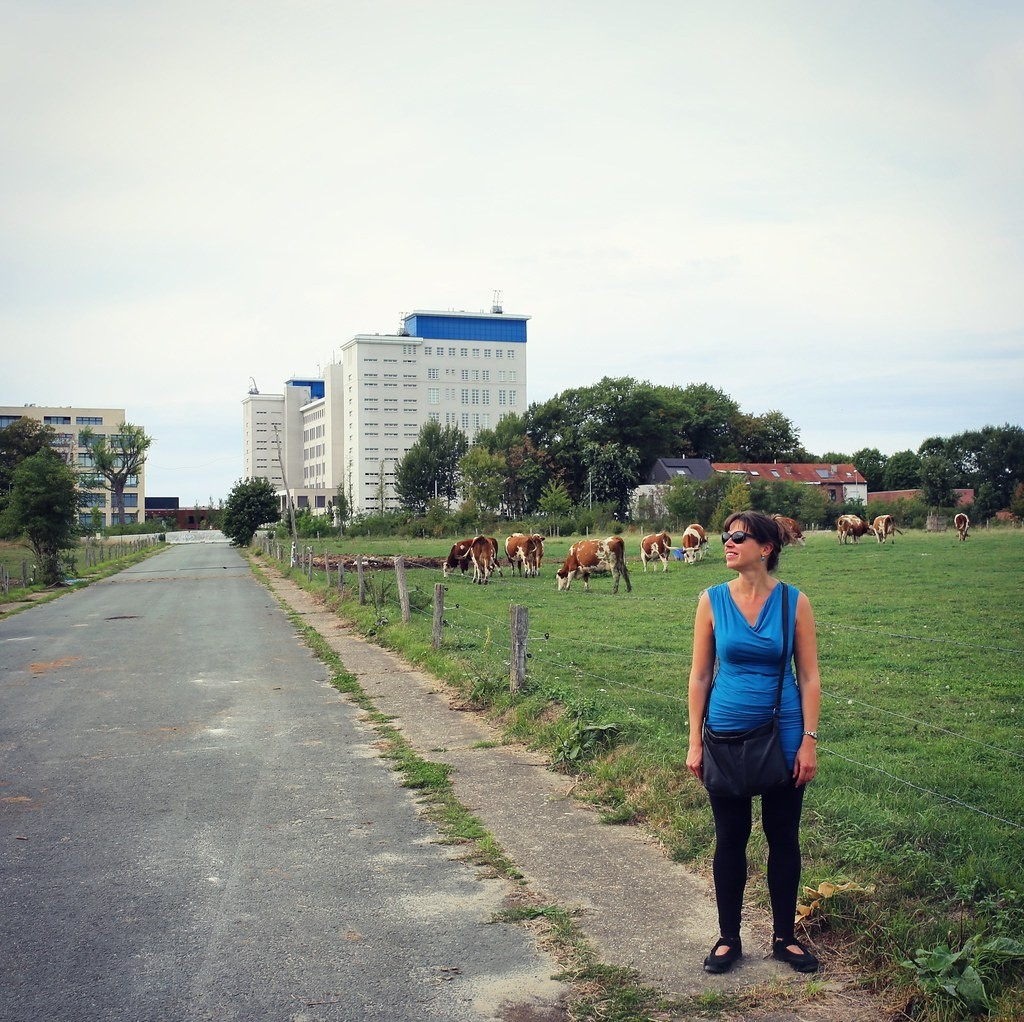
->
[703,708,790,796]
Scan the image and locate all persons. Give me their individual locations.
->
[686,511,821,974]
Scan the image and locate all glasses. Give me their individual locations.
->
[722,531,756,545]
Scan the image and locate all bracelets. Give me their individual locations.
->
[802,731,818,739]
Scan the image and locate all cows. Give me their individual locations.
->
[837,514,897,545]
[443,535,503,586]
[504,532,546,578]
[555,535,631,594]
[774,516,807,547]
[640,531,680,573]
[680,523,708,565]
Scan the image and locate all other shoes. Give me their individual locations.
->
[703,937,743,973]
[773,935,819,972]
